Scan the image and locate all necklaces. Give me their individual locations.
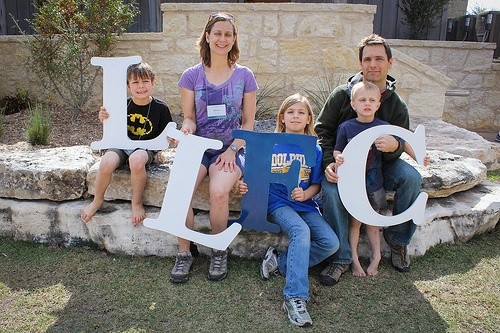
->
[128,96,152,140]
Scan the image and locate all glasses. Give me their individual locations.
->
[209,12,235,22]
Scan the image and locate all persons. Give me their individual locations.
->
[81,63,175,225]
[332,83,430,275]
[239,93,339,327]
[170,13,259,283]
[314,34,420,286]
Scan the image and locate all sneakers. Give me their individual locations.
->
[207,247,228,282]
[259,245,279,281]
[169,250,194,284]
[391,245,411,272]
[282,297,313,327]
[319,263,350,286]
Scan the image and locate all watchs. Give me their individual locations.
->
[229,145,238,154]
